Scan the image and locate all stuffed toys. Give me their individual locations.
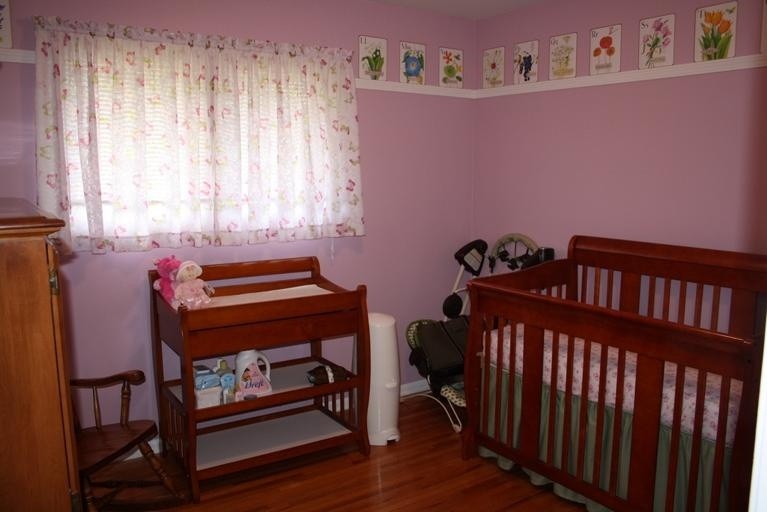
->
[174,260,215,309]
[151,254,180,304]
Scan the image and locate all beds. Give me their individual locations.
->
[457,236,766,512]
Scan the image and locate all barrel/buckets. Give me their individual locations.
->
[234,349,274,398]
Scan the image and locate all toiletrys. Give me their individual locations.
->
[212,358,222,373]
[215,360,235,401]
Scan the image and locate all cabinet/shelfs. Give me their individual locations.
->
[147,256,370,503]
[0,197,82,512]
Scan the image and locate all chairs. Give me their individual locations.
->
[71,369,179,511]
